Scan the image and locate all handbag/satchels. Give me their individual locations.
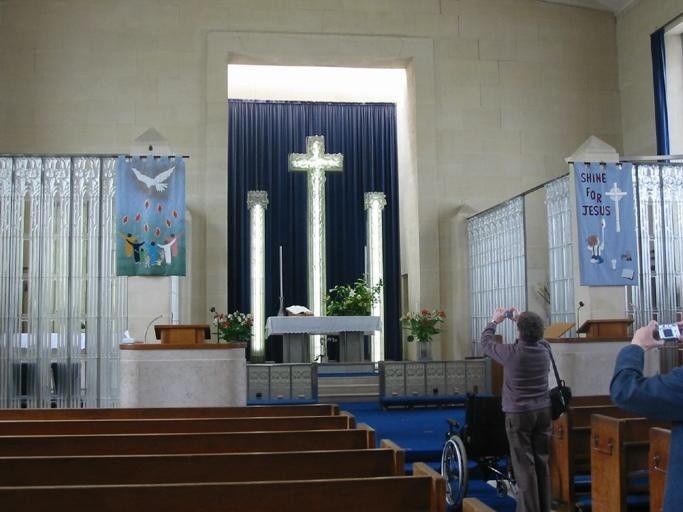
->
[549,385,572,421]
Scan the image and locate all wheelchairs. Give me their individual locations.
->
[440,384,519,508]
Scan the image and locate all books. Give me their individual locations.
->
[284,304,312,315]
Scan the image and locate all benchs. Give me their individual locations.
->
[538,391,672,511]
[1,402,499,512]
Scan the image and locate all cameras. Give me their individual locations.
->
[503,312,513,318]
[657,323,681,339]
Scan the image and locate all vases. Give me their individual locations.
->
[415,337,431,361]
[333,311,371,364]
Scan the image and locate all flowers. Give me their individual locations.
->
[394,306,449,345]
[319,273,386,318]
[210,309,253,347]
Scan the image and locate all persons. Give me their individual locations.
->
[479,306,556,512]
[606,318,683,512]
[116,227,185,270]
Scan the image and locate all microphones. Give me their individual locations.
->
[578,301,584,307]
